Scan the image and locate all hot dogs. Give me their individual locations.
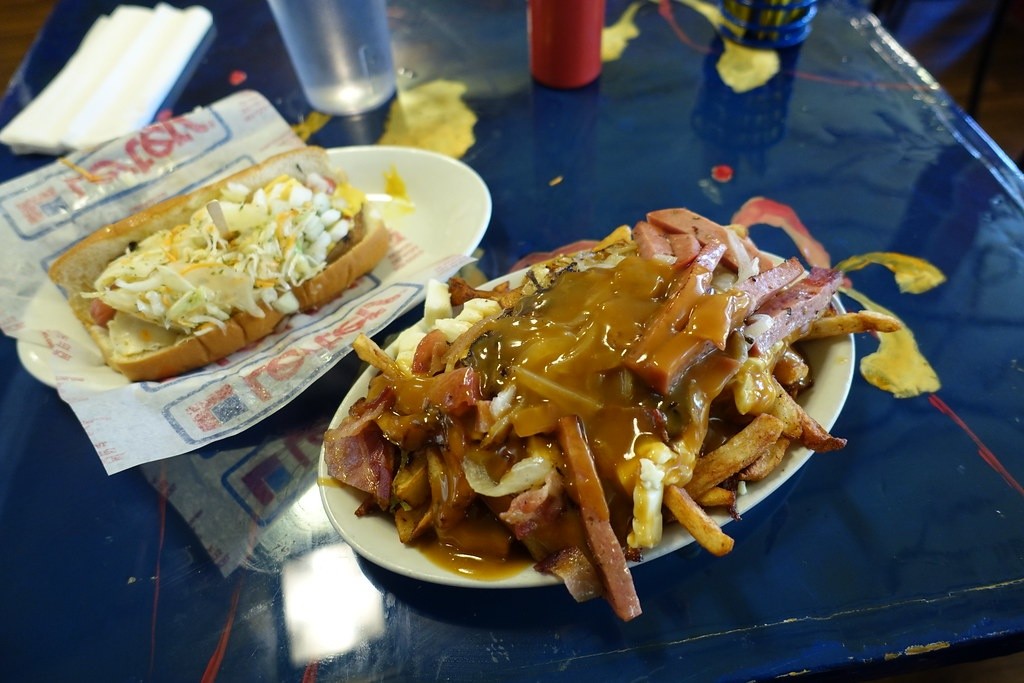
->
[49,144,390,381]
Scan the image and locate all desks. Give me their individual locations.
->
[0,0,1024,683]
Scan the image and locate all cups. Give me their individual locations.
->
[524,0,606,89]
[267,0,398,116]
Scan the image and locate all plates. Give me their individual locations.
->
[17,146,498,391]
[316,241,855,590]
